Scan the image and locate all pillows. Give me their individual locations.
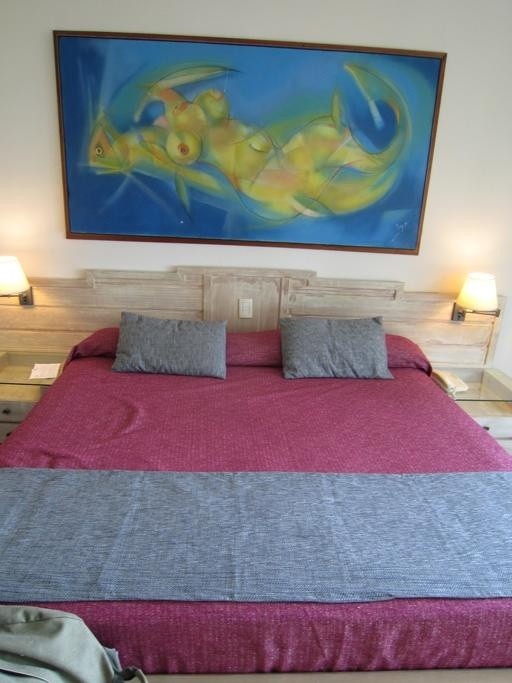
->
[277,316,395,380]
[111,311,228,379]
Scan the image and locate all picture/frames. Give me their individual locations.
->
[52,30,447,256]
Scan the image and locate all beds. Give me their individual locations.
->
[0,327,512,683]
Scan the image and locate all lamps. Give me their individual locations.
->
[0,255,33,305]
[451,271,501,323]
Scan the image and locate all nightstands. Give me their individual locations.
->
[0,351,69,445]
[428,367,512,458]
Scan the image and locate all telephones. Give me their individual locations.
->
[432,368,470,394]
